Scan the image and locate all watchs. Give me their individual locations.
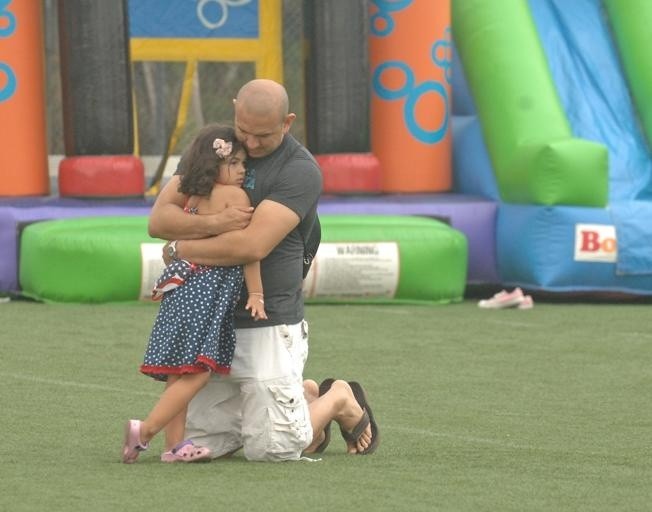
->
[168,240,176,260]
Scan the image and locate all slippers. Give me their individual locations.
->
[123,419,150,464]
[339,382,381,454]
[314,377,338,453]
[161,440,211,464]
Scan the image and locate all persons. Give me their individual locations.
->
[149,79,380,463]
[120,125,269,465]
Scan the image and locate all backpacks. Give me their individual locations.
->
[257,138,322,281]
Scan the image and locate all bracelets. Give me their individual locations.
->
[249,293,264,296]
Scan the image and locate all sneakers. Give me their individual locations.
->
[516,294,534,310]
[477,287,525,308]
